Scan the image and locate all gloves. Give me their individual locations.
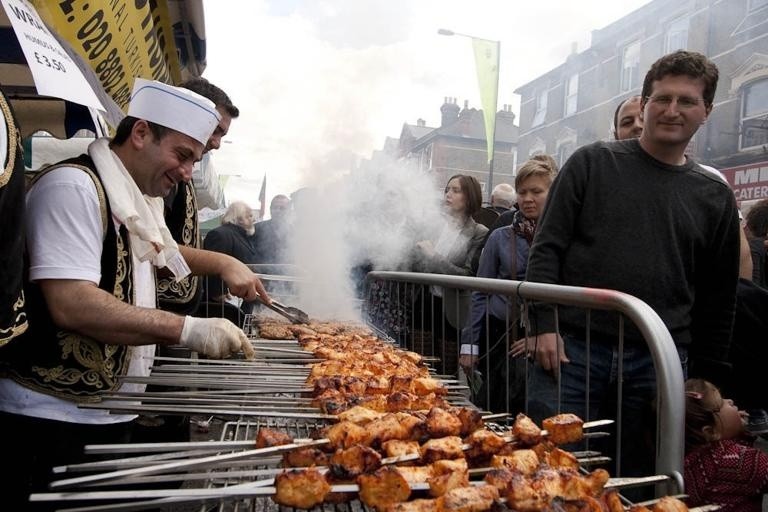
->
[178,315,255,360]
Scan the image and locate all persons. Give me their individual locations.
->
[0,77,254,512]
[130,74,268,462]
[652,379,768,512]
[520,51,741,504]
[251,193,290,273]
[460,155,558,425]
[614,95,768,448]
[345,177,516,379]
[202,197,259,301]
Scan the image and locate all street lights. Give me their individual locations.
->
[221,141,232,144]
[218,174,241,181]
[438,28,501,208]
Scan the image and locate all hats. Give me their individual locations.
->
[126,77,222,148]
